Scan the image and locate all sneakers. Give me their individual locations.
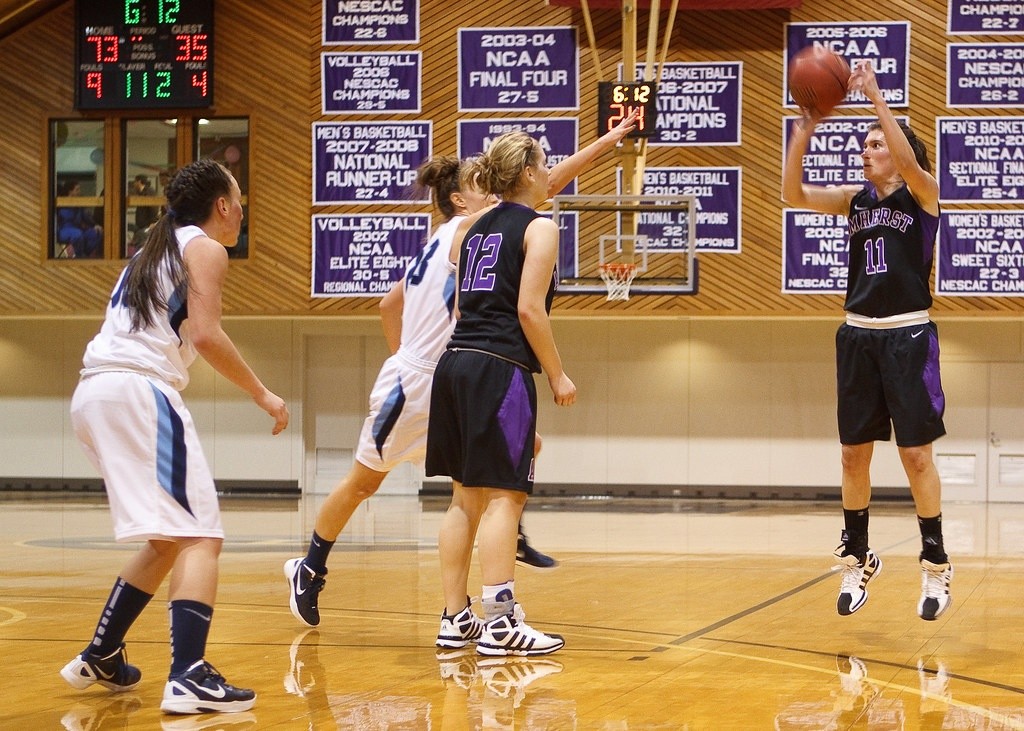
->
[285,557,328,628]
[60,643,141,694]
[833,547,883,615]
[434,595,486,648]
[917,551,954,620]
[476,602,565,656]
[159,658,256,713]
[515,525,557,570]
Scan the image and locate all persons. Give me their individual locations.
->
[781,59,956,621]
[284,104,642,629]
[60,159,288,716]
[423,129,579,657]
[55,175,160,259]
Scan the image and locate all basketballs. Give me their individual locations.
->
[789,48,851,111]
